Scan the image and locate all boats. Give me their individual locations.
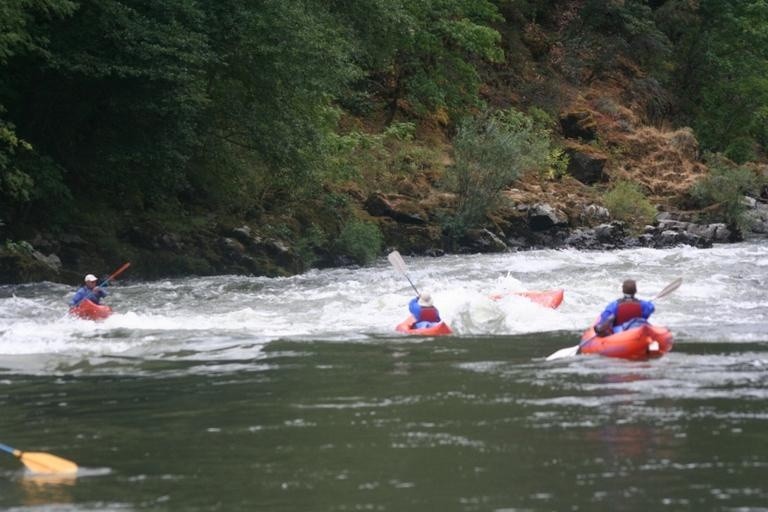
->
[397,315,453,339]
[579,311,675,361]
[67,297,112,321]
[491,289,566,311]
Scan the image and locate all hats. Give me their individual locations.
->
[622,280,635,293]
[417,293,433,307]
[84,274,97,282]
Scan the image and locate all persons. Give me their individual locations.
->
[594,278,655,337]
[68,274,109,307]
[406,294,440,328]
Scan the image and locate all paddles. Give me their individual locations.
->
[71,263,129,302]
[388,251,419,297]
[1,442,79,471]
[546,277,683,359]
